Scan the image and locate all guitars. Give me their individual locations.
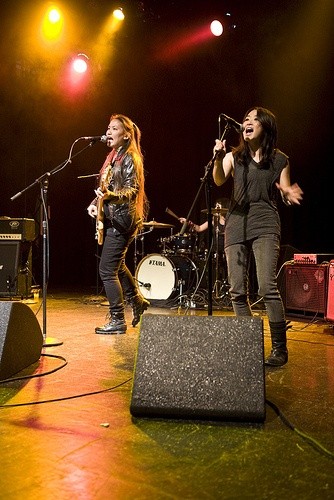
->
[94,164,114,247]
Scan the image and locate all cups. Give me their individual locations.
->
[34,291,40,301]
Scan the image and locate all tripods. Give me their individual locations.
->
[201,215,230,308]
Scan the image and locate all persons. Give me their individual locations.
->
[180,201,228,281]
[87,113,149,334]
[213,107,303,366]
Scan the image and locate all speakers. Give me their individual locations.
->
[0,239,33,297]
[0,300,44,380]
[283,265,328,314]
[129,313,266,424]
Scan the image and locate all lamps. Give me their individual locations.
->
[72,52,90,73]
[113,1,130,21]
[210,13,237,36]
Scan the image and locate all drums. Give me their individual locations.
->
[169,234,201,255]
[134,253,198,302]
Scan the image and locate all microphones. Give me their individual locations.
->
[220,114,245,133]
[143,283,151,287]
[81,135,107,142]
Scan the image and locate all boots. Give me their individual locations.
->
[265,321,293,366]
[95,303,126,334]
[127,291,150,325]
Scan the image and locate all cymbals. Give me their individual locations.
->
[200,208,230,213]
[141,222,176,228]
